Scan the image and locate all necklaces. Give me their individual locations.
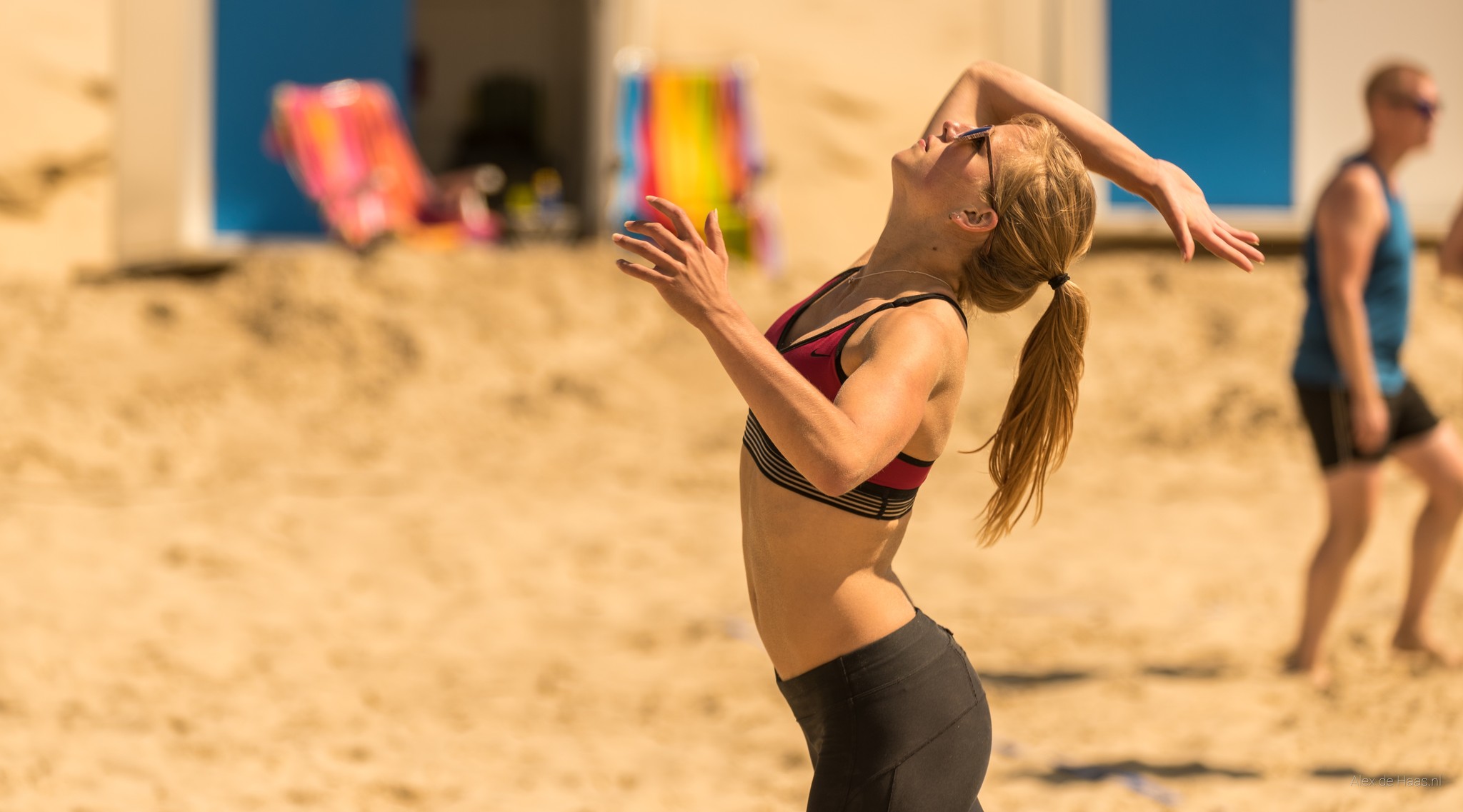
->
[848,269,952,289]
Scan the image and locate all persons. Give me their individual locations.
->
[610,59,1262,812]
[1276,62,1463,672]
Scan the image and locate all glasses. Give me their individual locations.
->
[957,123,997,213]
[1393,103,1437,119]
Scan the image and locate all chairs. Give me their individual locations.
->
[613,63,775,270]
[264,77,507,249]
